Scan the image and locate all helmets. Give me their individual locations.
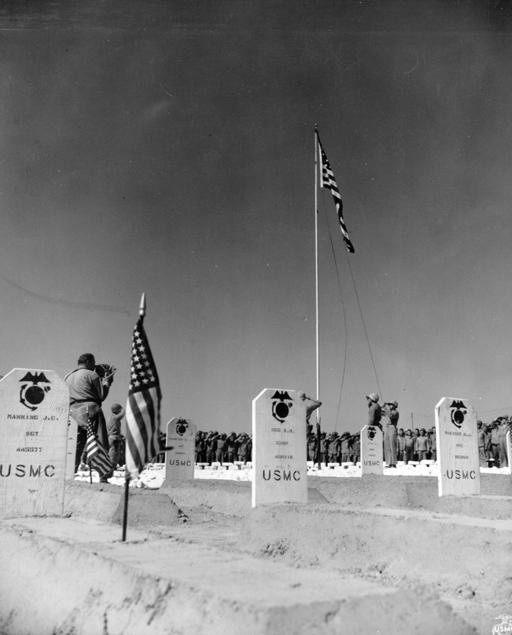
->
[386,399,398,408]
[111,403,122,412]
[366,392,378,402]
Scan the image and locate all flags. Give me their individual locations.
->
[125,316,162,480]
[86,412,117,480]
[318,138,355,253]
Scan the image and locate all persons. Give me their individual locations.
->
[119,431,253,466]
[108,404,125,470]
[476,414,512,468]
[300,394,438,468]
[63,353,114,484]
